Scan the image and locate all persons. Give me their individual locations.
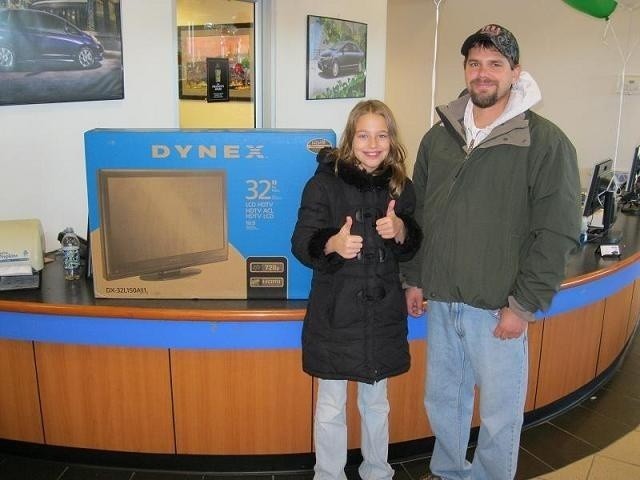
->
[398,24,584,480]
[290,99,425,480]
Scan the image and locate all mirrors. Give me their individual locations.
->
[170,0,263,129]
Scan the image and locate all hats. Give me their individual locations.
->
[461,23,520,65]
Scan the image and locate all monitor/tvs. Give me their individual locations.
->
[621,144,640,213]
[98,168,228,282]
[583,159,624,244]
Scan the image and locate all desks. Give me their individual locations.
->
[0,213,640,473]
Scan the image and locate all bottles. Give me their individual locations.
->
[61,228,81,280]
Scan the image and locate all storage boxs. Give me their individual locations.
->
[83,127,337,301]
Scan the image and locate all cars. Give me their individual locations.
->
[317,40,365,77]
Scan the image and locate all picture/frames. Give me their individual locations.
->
[0,0,124,106]
[178,23,254,102]
[306,15,368,100]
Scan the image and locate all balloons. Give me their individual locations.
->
[562,0,618,21]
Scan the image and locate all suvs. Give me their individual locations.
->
[0,8,104,73]
